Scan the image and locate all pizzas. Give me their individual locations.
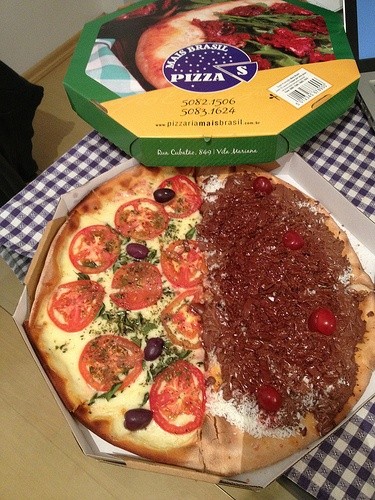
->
[134,1,338,90]
[22,161,375,478]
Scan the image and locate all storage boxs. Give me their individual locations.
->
[12,1,374,490]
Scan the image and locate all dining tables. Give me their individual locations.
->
[1,101,375,500]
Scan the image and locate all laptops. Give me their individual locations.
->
[341,0,375,133]
